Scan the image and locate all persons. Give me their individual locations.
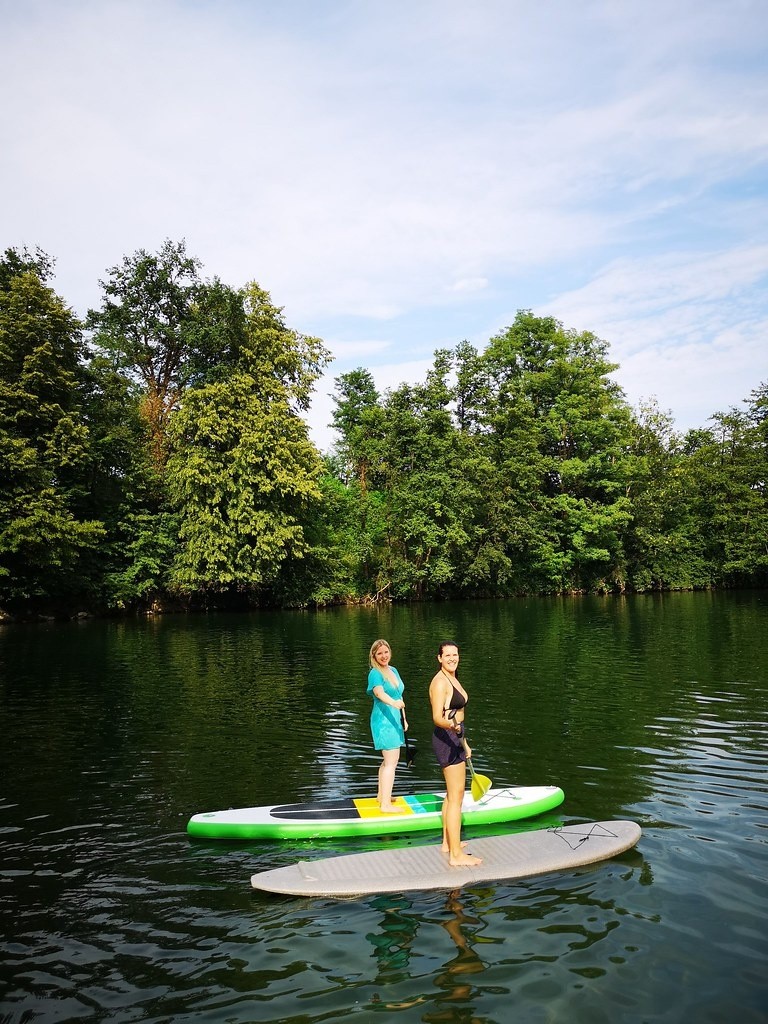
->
[429,642,483,867]
[366,638,408,813]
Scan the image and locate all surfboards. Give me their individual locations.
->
[187,784,567,842]
[251,818,644,897]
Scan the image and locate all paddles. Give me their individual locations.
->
[448,709,493,802]
[400,689,418,769]
